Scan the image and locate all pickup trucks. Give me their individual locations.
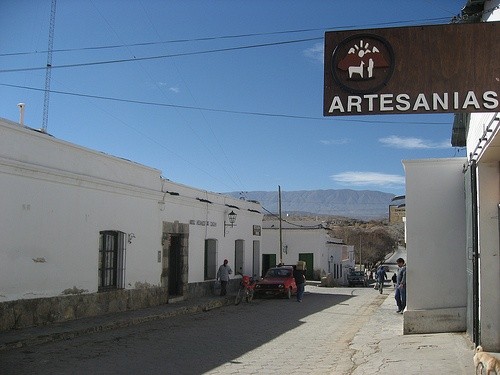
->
[348,271,368,287]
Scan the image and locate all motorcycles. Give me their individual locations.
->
[233,272,259,306]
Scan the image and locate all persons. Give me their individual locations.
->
[216,259,232,296]
[294,261,306,303]
[376,267,387,294]
[392,258,406,313]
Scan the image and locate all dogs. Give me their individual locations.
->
[473,345,500,375]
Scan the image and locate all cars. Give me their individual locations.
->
[255,268,297,299]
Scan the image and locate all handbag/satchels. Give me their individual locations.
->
[374,282,379,290]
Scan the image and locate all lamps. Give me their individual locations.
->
[225,210,237,228]
[328,256,334,263]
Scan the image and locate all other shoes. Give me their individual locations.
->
[400,309,404,314]
[397,306,402,312]
